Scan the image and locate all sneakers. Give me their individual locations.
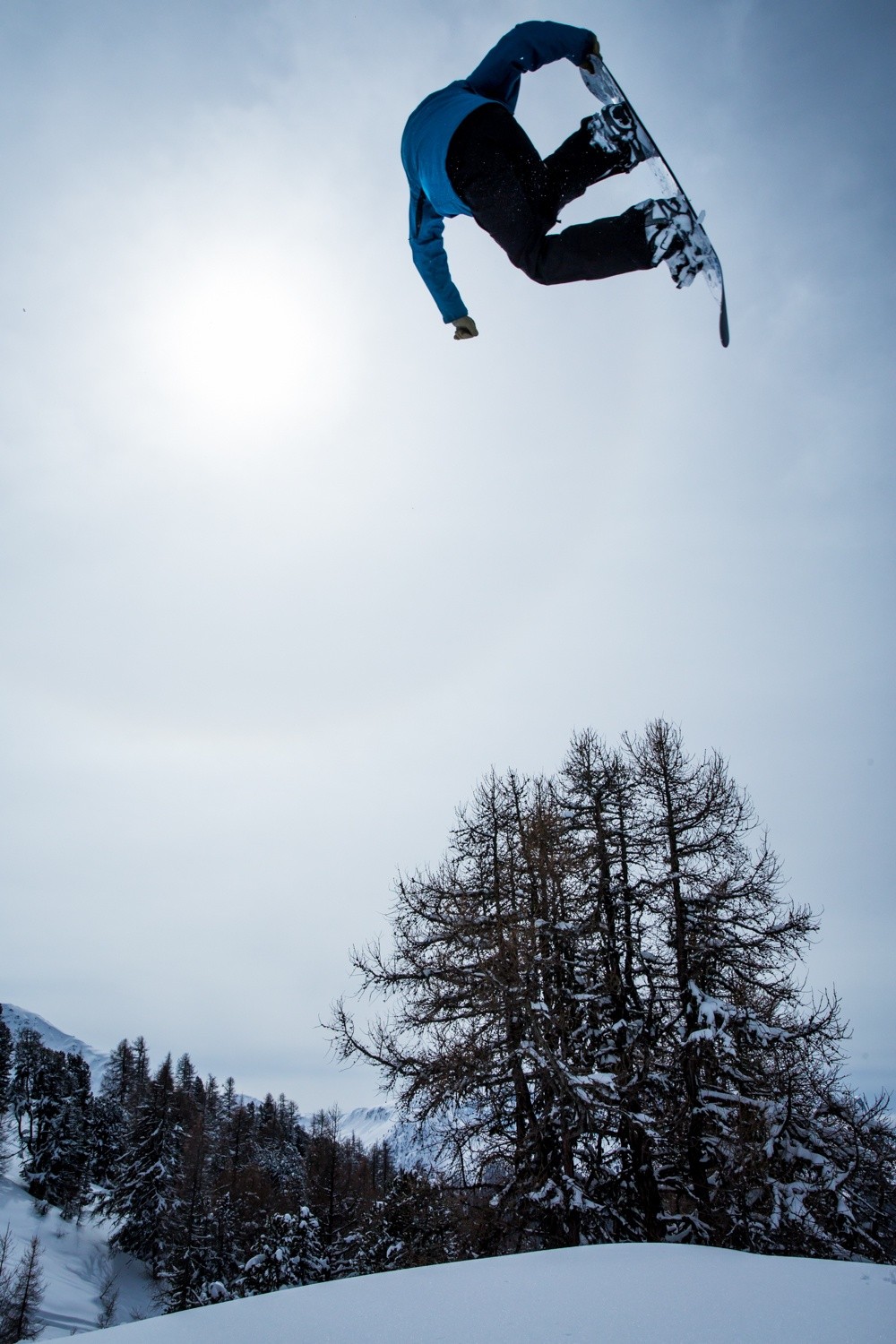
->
[636,194,706,290]
[586,102,646,176]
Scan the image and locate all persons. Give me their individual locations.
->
[399,19,704,340]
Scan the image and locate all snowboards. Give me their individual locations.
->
[578,54,731,348]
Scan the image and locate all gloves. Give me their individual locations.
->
[451,316,479,341]
[579,38,600,75]
